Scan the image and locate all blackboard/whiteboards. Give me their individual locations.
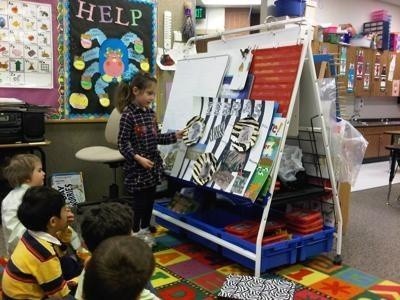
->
[0,0,158,124]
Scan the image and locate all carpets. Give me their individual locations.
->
[1,223,399,300]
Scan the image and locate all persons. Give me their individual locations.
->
[0,154,79,260]
[0,186,82,300]
[75,201,162,300]
[82,235,156,300]
[115,72,186,237]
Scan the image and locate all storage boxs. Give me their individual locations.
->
[152,201,337,275]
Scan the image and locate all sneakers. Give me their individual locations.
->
[132,227,157,247]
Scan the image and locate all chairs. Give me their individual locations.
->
[75,107,135,215]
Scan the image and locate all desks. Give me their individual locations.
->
[0,140,52,186]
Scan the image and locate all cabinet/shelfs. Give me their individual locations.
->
[311,41,400,98]
[355,125,400,164]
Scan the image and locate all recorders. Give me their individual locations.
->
[0,102,44,145]
